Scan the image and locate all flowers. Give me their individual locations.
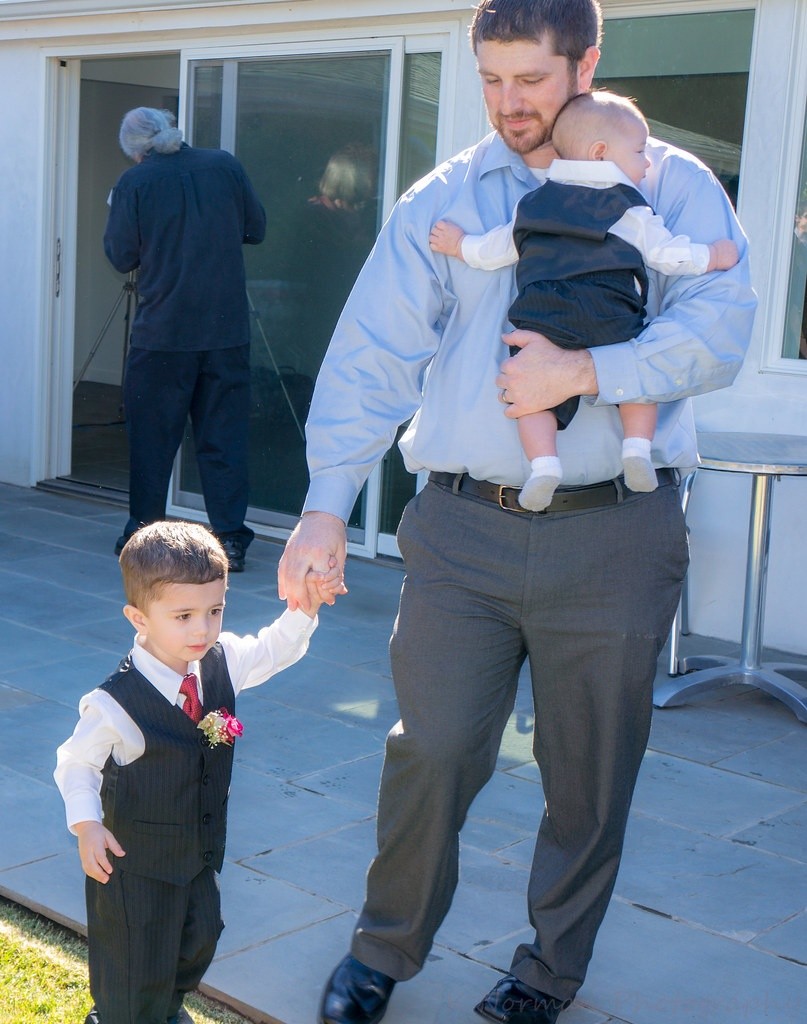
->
[197,707,243,748]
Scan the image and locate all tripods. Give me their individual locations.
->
[71,270,138,420]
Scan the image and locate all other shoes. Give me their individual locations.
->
[114,537,131,557]
[217,537,246,573]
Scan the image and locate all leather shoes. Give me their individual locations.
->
[475,973,569,1024]
[320,954,396,1024]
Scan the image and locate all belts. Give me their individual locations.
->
[428,467,674,514]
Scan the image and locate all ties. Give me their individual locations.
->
[181,674,203,725]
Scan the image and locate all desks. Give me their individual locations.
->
[653,430,806,725]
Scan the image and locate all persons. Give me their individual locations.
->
[53,520,346,1023]
[103,108,267,572]
[278,1,760,1024]
[429,92,738,512]
